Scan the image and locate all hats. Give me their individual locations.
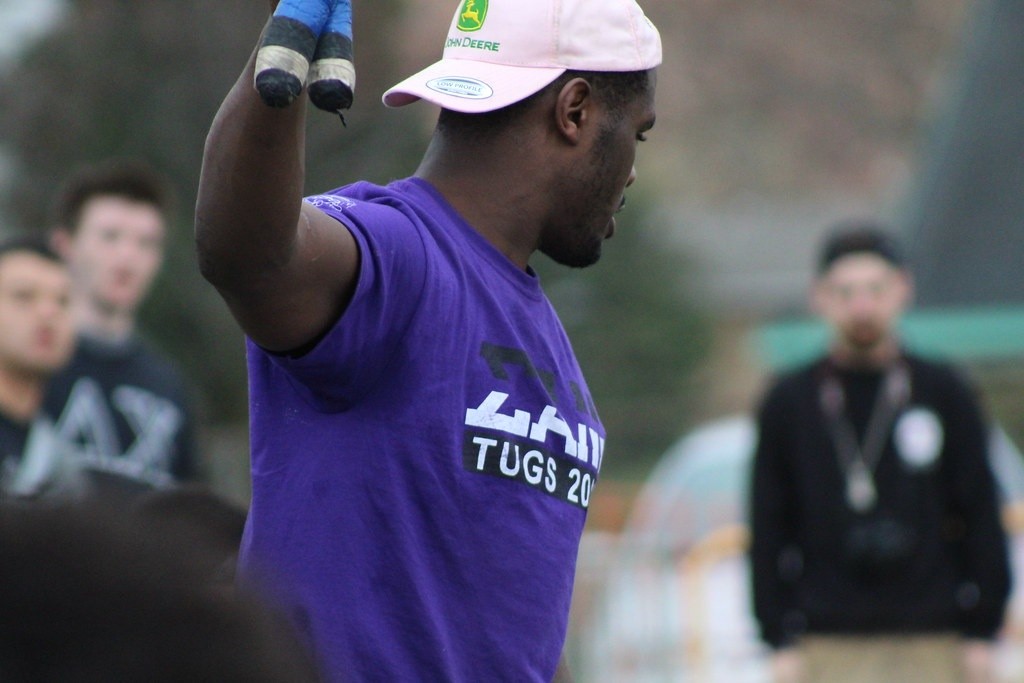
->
[381,0,662,117]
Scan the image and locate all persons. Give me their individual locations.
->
[0,463,321,682]
[747,222,1016,652]
[196,2,662,678]
[32,166,212,494]
[0,239,100,496]
